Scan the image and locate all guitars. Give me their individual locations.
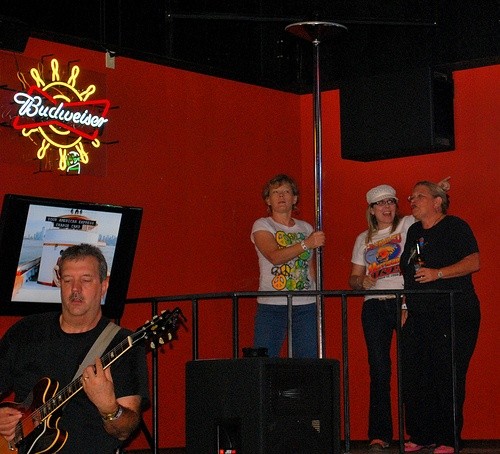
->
[0,307,181,454]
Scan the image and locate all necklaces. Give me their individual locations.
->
[433,214,444,226]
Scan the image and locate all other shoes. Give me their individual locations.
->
[368,439,390,450]
[434,445,454,454]
[403,441,436,452]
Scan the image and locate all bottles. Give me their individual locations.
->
[413,241,426,279]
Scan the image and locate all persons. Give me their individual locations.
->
[249,174,326,360]
[347,175,453,452]
[0,243,151,454]
[398,180,481,454]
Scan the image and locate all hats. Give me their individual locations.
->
[366,185,398,204]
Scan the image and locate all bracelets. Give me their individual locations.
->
[401,303,408,310]
[301,240,308,251]
[99,403,124,421]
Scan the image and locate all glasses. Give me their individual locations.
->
[373,200,396,206]
[408,195,437,202]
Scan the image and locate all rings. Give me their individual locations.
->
[421,275,423,279]
[84,377,89,381]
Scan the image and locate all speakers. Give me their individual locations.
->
[340,65,455,161]
[184,358,341,454]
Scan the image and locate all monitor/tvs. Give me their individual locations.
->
[0,195,143,318]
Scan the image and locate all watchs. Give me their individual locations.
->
[437,269,443,279]
